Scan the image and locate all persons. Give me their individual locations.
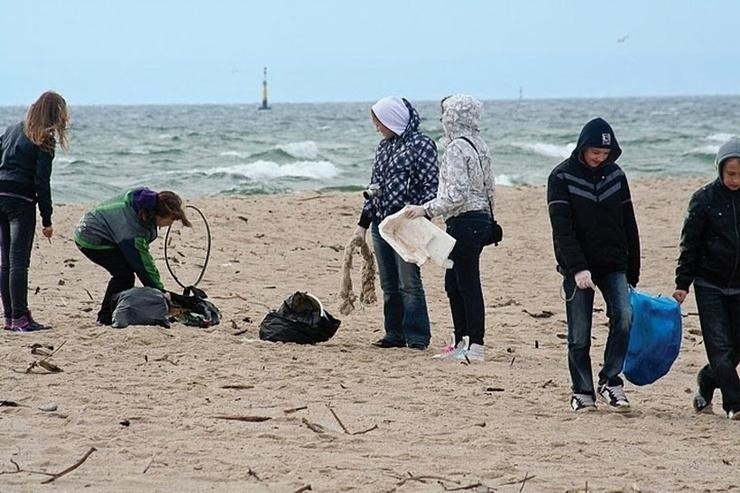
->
[404,94,495,366]
[73,185,182,325]
[548,118,642,413]
[0,90,66,330]
[352,96,437,350]
[673,139,740,420]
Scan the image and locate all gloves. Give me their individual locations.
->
[573,270,597,292]
[354,225,366,242]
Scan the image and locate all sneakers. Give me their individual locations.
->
[446,344,486,363]
[596,380,632,413]
[726,406,739,416]
[693,395,713,416]
[11,314,45,333]
[571,392,599,413]
[409,344,427,350]
[430,341,470,360]
[4,316,12,328]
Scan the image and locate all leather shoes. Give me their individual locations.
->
[372,336,406,348]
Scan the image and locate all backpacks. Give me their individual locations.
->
[449,136,503,247]
